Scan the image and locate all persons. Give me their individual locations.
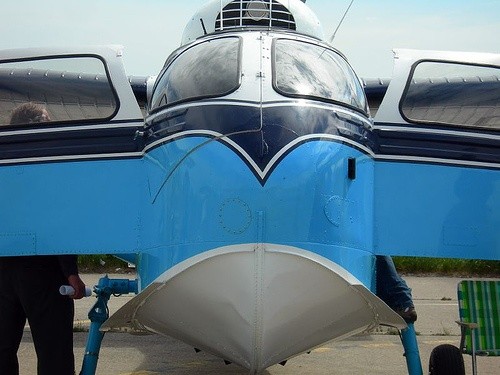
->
[0,101,87,374]
[373,250,420,326]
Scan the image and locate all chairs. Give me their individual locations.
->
[454,279,500,375]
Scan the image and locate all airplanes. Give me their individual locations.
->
[0,0,500,375]
